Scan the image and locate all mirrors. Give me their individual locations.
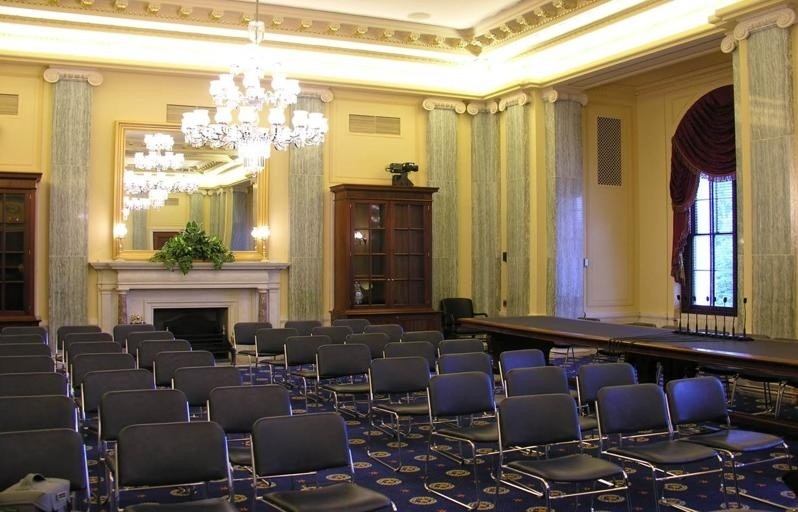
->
[113,120,270,262]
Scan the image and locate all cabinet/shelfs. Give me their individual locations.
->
[330,183,440,312]
[0,171,43,321]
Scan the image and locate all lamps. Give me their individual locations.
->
[180,1,328,184]
[123,132,205,206]
[122,169,160,221]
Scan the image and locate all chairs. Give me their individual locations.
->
[441,298,488,339]
